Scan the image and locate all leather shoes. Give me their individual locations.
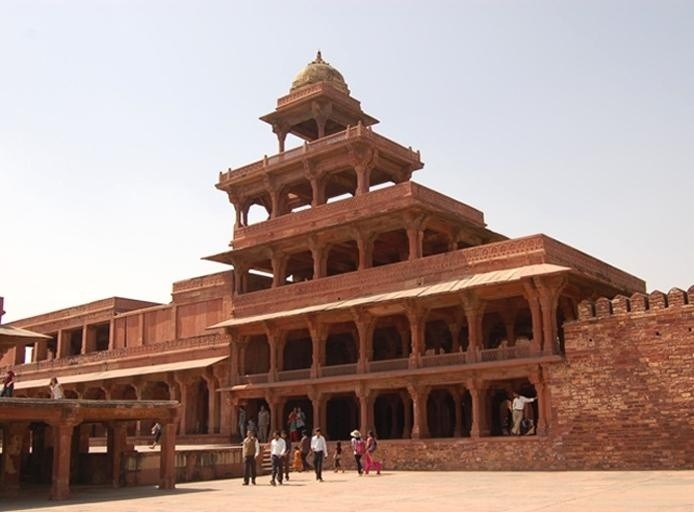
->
[241,481,255,485]
[268,479,282,486]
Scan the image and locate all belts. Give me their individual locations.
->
[514,409,522,411]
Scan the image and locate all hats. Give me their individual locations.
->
[350,429,362,438]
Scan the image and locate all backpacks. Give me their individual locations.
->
[355,439,366,454]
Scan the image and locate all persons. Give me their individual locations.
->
[350,429,365,475]
[0,370,15,397]
[332,440,345,472]
[47,376,65,399]
[149,422,160,449]
[310,427,328,482]
[506,389,537,436]
[237,404,313,487]
[363,430,381,475]
